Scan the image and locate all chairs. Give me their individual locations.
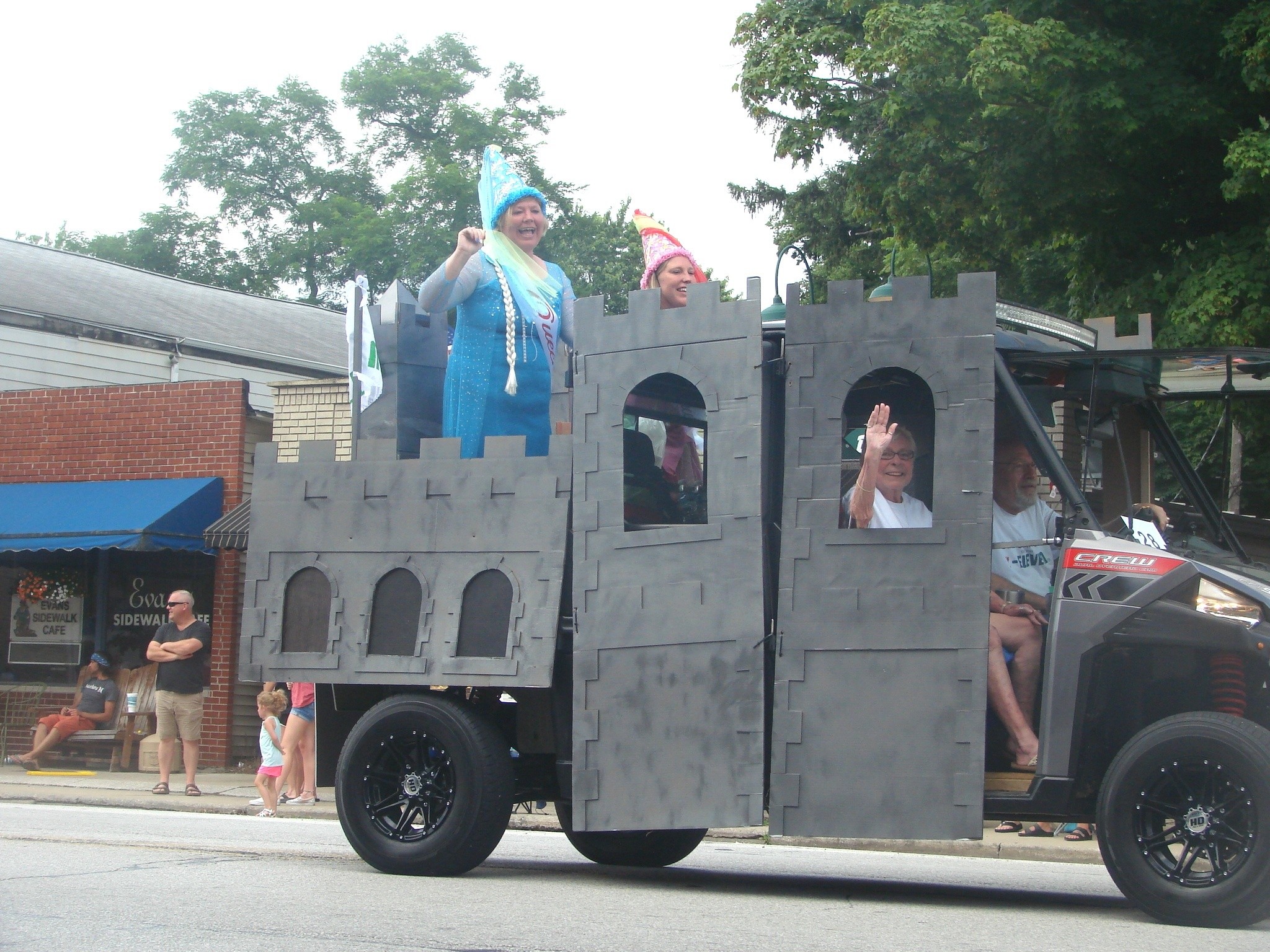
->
[0,682,47,766]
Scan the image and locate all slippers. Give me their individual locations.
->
[186,784,201,796]
[995,820,1023,832]
[10,754,40,771]
[1010,754,1037,773]
[1064,827,1092,841]
[152,781,169,794]
[1018,823,1054,837]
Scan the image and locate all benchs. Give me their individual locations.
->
[30,662,159,773]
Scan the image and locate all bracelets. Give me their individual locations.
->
[856,479,874,492]
[1001,601,1011,613]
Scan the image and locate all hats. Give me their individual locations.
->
[485,144,546,231]
[633,208,694,292]
[623,429,663,486]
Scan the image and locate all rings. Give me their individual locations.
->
[866,424,872,428]
[873,422,887,426]
[888,432,893,435]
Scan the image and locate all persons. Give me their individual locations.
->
[418,144,576,458]
[146,590,211,796]
[850,402,1049,773]
[994,819,1093,841]
[249,682,320,818]
[632,209,709,499]
[992,432,1169,639]
[8,650,116,770]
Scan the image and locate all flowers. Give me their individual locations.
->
[15,568,85,603]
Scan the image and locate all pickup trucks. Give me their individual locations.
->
[240,271,1270,923]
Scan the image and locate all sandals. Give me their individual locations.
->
[256,808,277,818]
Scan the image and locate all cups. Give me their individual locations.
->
[126,693,138,713]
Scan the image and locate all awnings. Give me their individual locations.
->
[203,496,251,552]
[0,475,224,558]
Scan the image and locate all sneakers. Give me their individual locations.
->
[249,797,281,806]
[286,795,315,806]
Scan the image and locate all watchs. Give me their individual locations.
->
[1132,502,1139,507]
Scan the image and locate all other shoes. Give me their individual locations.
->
[279,792,295,803]
[315,799,320,801]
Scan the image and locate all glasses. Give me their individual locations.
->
[995,461,1037,471]
[166,602,189,607]
[881,450,914,460]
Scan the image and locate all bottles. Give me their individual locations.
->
[1045,587,1054,615]
